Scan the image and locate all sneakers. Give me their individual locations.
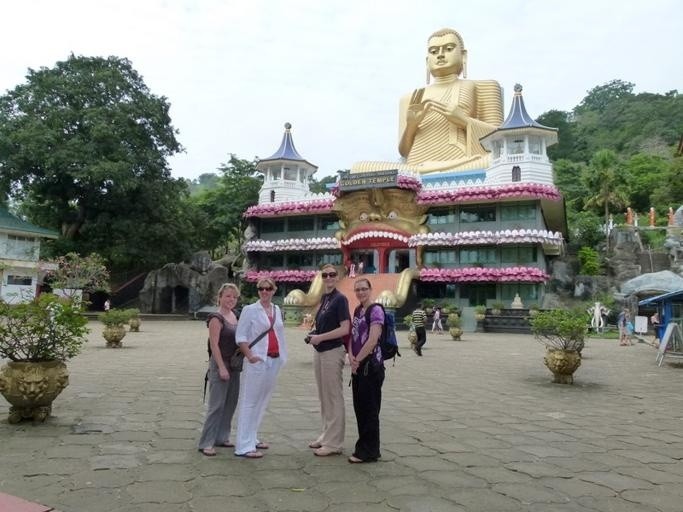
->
[414,331,444,356]
[618,340,660,346]
[314,446,343,456]
[348,452,381,464]
[308,441,321,448]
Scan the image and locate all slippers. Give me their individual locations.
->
[255,439,269,449]
[197,445,216,456]
[235,448,263,459]
[216,439,235,447]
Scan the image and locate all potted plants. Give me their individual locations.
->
[0,291,88,424]
[531,309,593,385]
[401,298,501,340]
[124,309,140,332]
[99,307,131,349]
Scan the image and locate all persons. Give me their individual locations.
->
[232,277,287,459]
[193,281,244,457]
[410,301,429,359]
[617,307,629,345]
[391,29,505,172]
[393,255,399,273]
[341,256,369,275]
[102,296,112,314]
[650,312,660,345]
[429,306,438,333]
[434,307,443,333]
[512,291,521,306]
[622,316,633,346]
[346,277,389,465]
[304,263,351,458]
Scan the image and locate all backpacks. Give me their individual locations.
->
[204,308,240,381]
[354,303,398,360]
[341,317,352,352]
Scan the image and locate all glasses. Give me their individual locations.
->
[258,286,274,292]
[321,272,338,279]
[354,287,370,292]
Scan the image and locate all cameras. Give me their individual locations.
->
[304,329,321,344]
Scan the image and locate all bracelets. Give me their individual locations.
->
[355,359,363,364]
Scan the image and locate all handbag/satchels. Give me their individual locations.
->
[359,354,380,379]
[229,349,244,372]
